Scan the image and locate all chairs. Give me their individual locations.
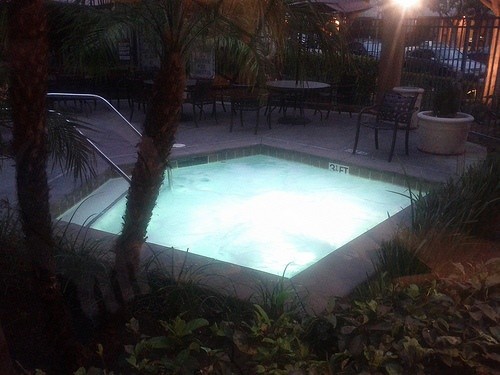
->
[352,89,419,163]
[44,64,360,135]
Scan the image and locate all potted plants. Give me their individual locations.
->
[415,81,475,156]
